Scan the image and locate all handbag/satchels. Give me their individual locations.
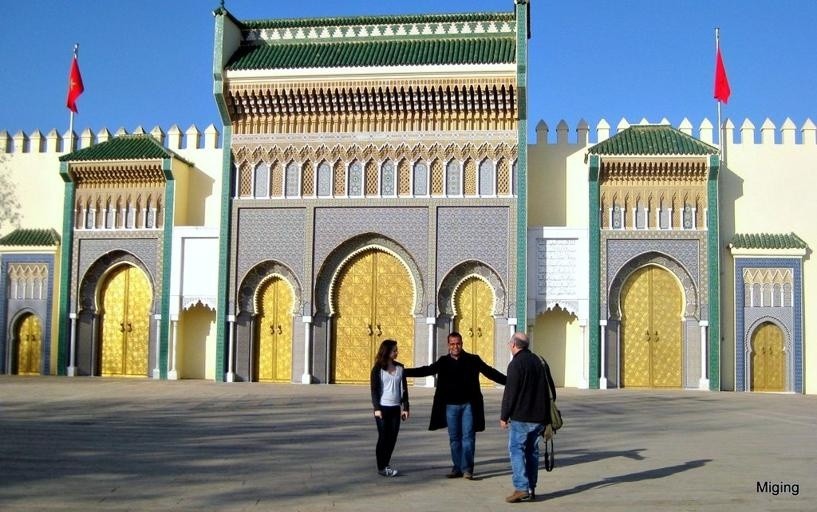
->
[541,403,563,441]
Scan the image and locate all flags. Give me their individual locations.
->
[66,52,85,113]
[713,38,731,105]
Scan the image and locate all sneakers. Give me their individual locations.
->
[463,473,473,480]
[446,470,462,478]
[529,488,536,501]
[505,491,528,503]
[378,467,399,477]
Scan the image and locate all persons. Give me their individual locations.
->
[370,340,410,479]
[402,330,506,480]
[499,330,557,502]
